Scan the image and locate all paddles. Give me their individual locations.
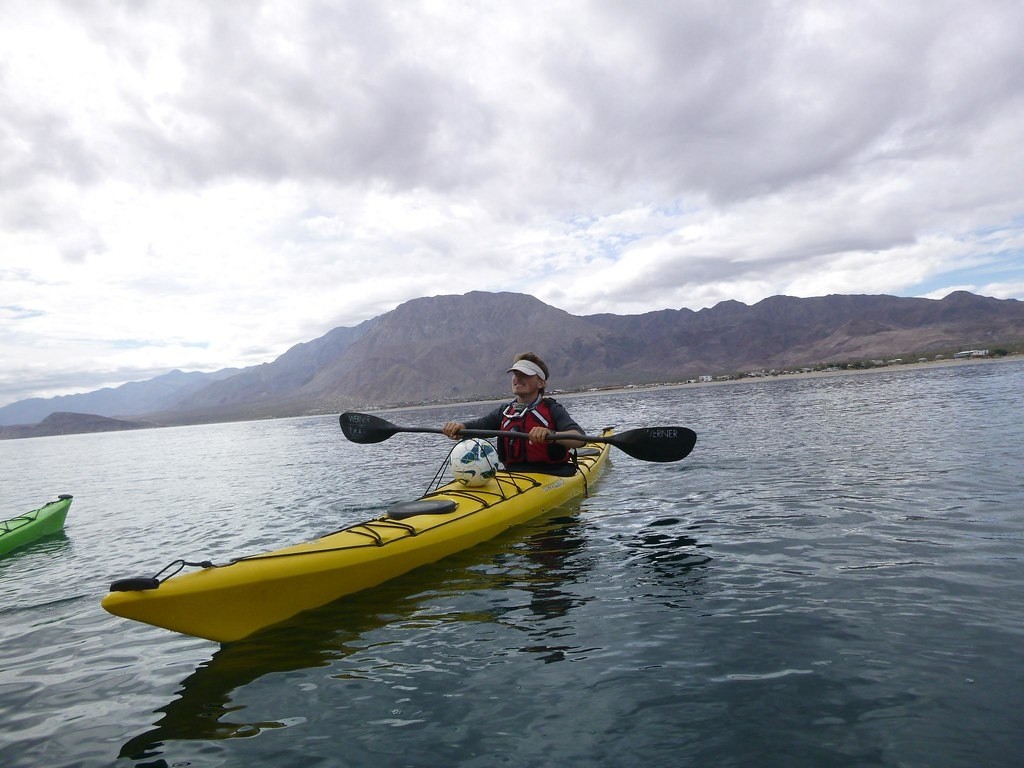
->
[337,410,699,463]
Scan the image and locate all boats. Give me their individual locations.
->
[1,494,73,556]
[100,426,613,642]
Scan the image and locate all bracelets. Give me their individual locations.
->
[548,430,556,444]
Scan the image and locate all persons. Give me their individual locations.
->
[442,353,586,464]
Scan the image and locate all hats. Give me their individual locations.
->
[506,359,546,381]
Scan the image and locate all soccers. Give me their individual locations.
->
[449,436,500,487]
